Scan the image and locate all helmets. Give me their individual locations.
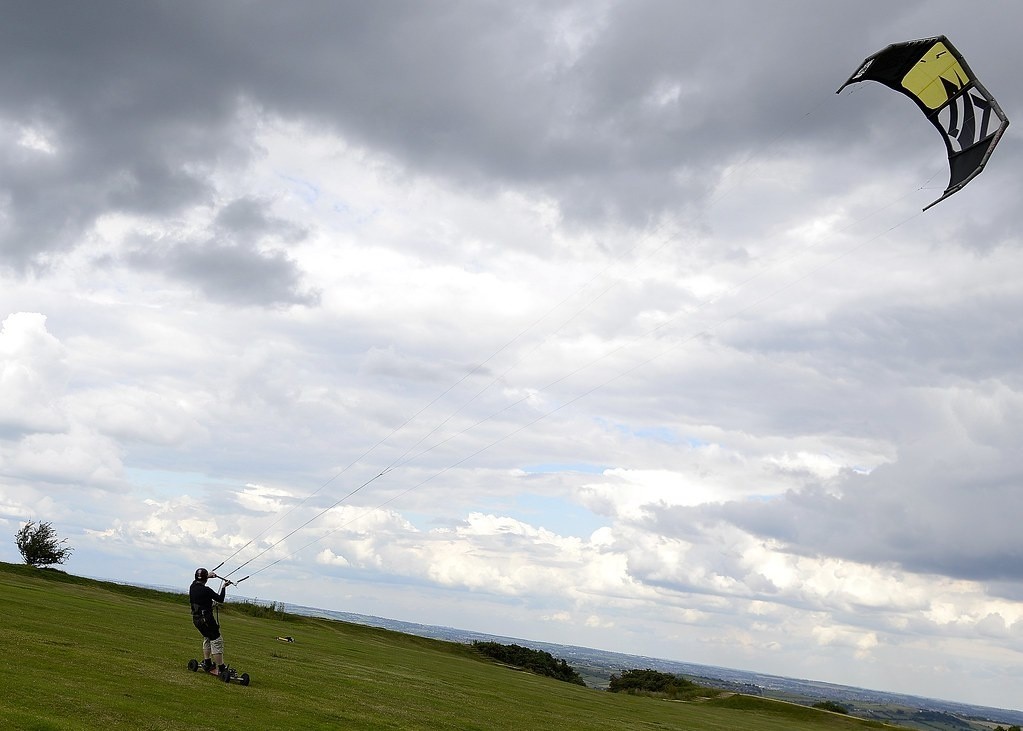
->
[194,569,208,583]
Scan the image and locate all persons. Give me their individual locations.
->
[189,568,231,672]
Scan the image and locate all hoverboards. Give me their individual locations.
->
[186,658,250,687]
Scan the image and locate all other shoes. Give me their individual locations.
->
[205,657,215,673]
[218,663,226,673]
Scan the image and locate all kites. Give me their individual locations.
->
[836,34,1011,212]
